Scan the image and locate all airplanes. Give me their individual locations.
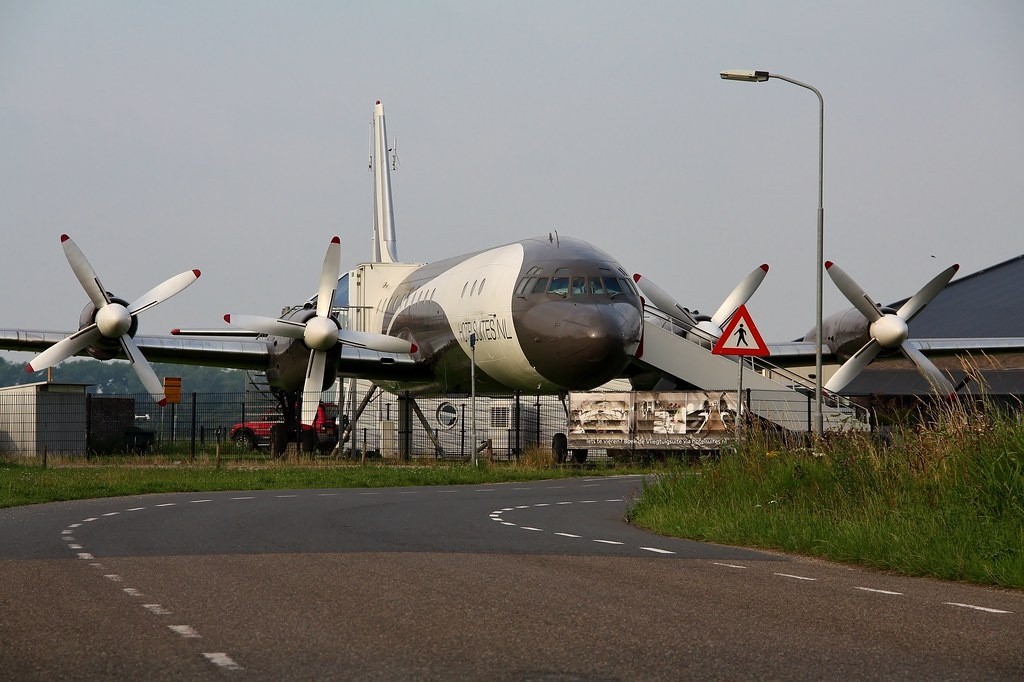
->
[1,98,1022,460]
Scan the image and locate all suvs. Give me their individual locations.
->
[228,400,351,457]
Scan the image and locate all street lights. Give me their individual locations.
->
[719,66,825,456]
